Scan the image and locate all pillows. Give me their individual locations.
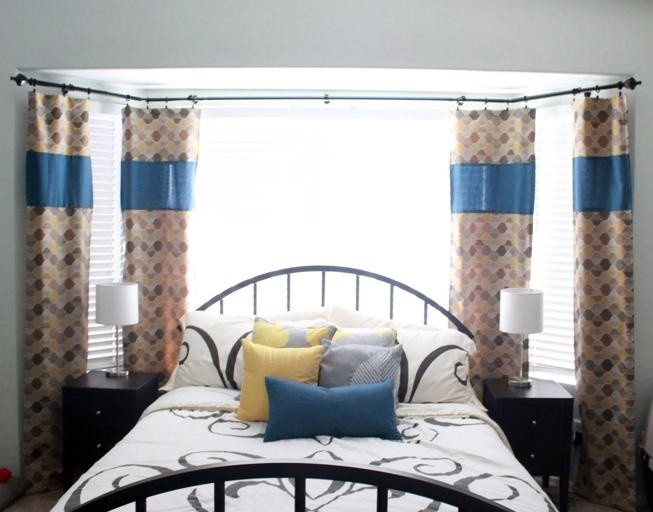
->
[163,306,475,443]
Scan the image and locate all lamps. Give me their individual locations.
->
[496,285,546,384]
[92,281,141,382]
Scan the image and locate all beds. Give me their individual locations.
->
[51,264,558,512]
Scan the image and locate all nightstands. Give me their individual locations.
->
[54,366,162,494]
[482,376,575,512]
